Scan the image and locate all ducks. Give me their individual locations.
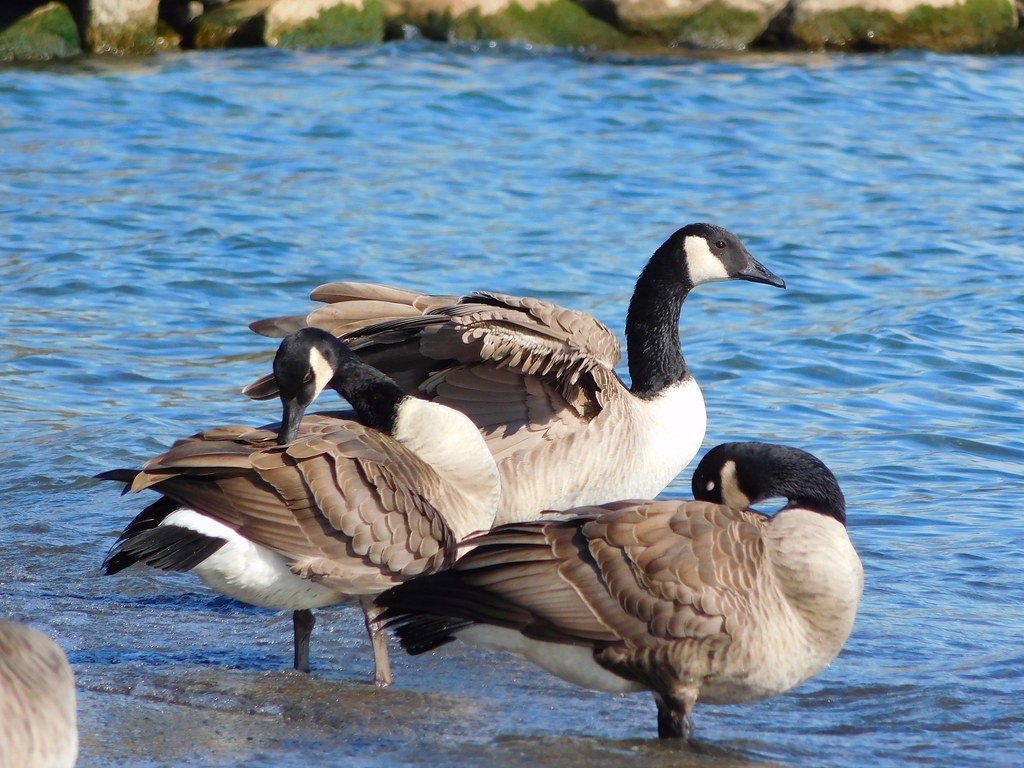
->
[241,223,785,530]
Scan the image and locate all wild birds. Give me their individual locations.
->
[365,441,864,740]
[0,618,79,768]
[92,327,501,687]
